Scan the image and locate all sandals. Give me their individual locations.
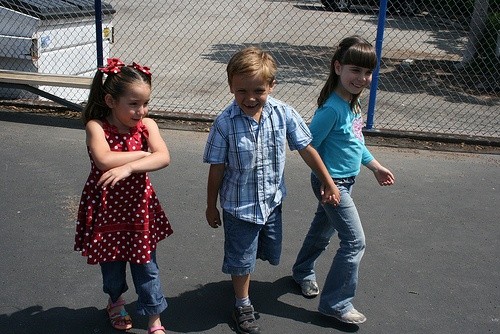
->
[107,297,132,329]
[148,326,166,334]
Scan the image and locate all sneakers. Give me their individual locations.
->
[293,274,319,295]
[233,303,260,334]
[318,307,367,323]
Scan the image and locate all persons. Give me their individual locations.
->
[292,36,395,325]
[203,47,340,334]
[73,58,174,334]
